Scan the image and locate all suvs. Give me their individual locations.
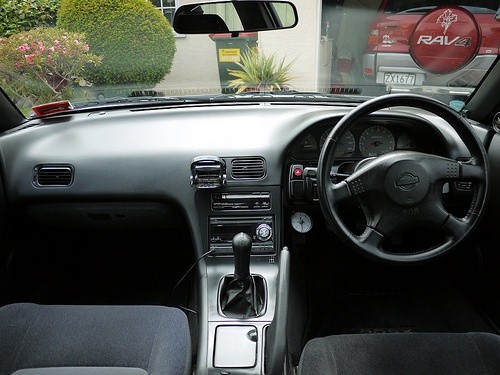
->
[359,0,500,112]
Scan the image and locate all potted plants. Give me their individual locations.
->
[225,40,299,95]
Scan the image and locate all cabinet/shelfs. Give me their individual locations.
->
[321,38,334,92]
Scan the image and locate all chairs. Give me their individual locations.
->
[296,332,500,375]
[0,302,193,375]
